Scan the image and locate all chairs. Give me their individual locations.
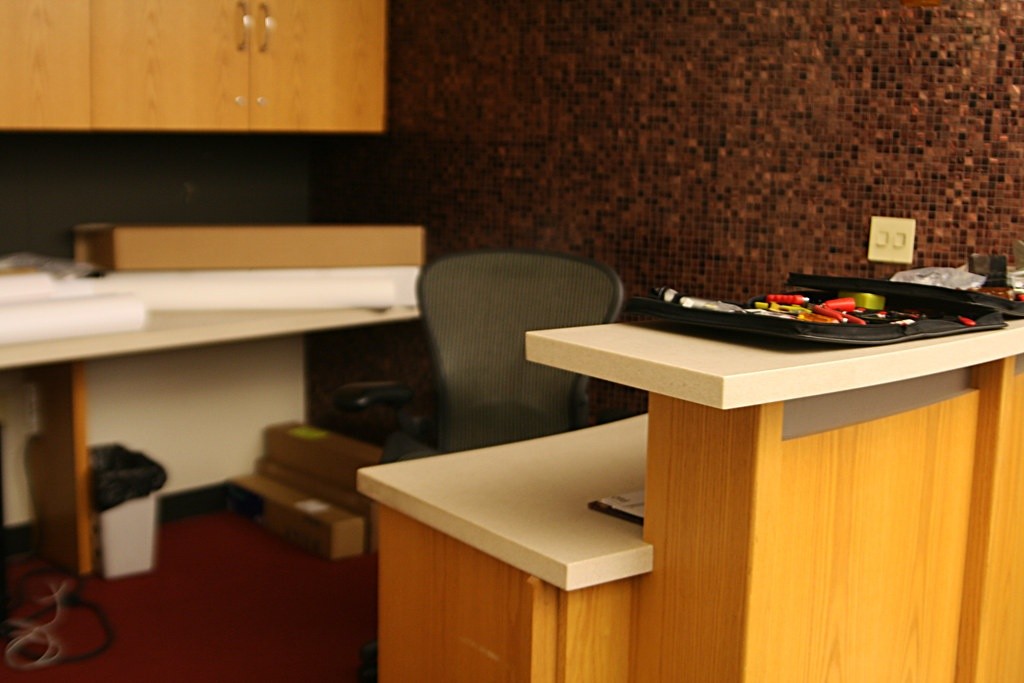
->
[381,248,624,463]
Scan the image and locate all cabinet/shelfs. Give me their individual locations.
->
[0,0,389,136]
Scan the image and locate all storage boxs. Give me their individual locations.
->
[78,221,424,271]
[229,428,382,560]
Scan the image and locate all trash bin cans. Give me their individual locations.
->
[88,444,166,579]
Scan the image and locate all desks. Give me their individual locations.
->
[359,317,1024,683]
[0,307,417,571]
